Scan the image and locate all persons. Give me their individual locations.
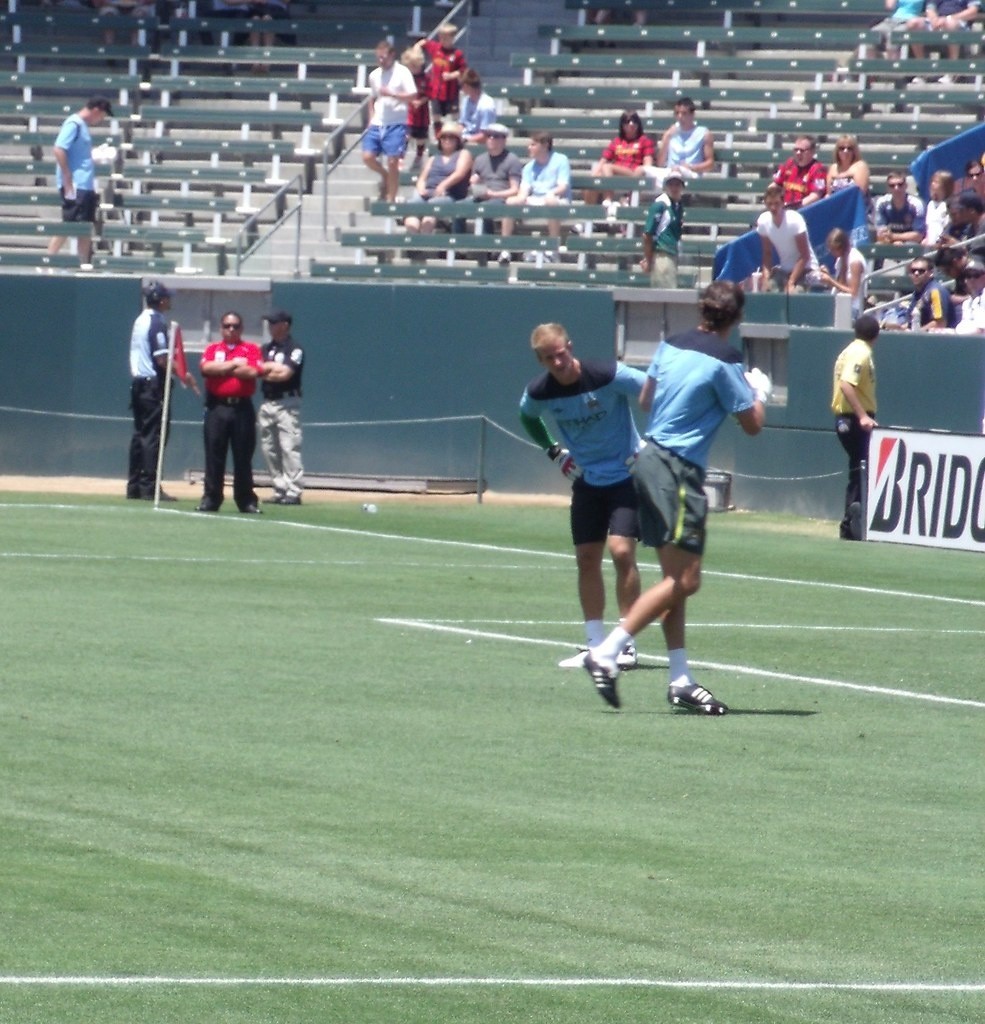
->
[768,133,869,211]
[397,23,468,170]
[405,122,572,263]
[258,310,305,503]
[756,187,821,294]
[518,324,649,669]
[593,9,648,24]
[571,98,715,233]
[195,312,265,515]
[34,0,293,74]
[48,97,114,256]
[855,0,985,84]
[639,172,686,289]
[583,279,772,715]
[875,161,985,335]
[362,40,417,202]
[126,281,197,502]
[459,68,498,145]
[831,316,879,540]
[819,227,869,325]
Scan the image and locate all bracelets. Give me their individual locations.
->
[547,445,561,460]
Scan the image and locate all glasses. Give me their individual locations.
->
[963,272,982,279]
[888,180,907,189]
[220,321,243,331]
[908,266,928,276]
[794,147,807,153]
[969,170,984,178]
[838,145,855,152]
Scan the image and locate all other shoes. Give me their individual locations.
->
[243,503,264,515]
[141,489,178,500]
[277,494,303,506]
[848,500,863,541]
[195,503,217,511]
[263,494,286,504]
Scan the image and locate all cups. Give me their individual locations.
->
[751,273,763,292]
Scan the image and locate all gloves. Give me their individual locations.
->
[746,368,773,407]
[548,444,580,481]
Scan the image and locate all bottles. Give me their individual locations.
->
[911,307,921,333]
[362,503,376,513]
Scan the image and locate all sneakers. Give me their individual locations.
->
[614,646,640,669]
[667,679,731,716]
[581,646,622,709]
[559,645,592,672]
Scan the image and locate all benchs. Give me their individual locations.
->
[0,0,985,306]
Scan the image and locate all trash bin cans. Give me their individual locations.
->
[701,470,731,512]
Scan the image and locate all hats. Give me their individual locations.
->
[435,123,470,141]
[85,96,116,118]
[259,309,293,324]
[140,278,175,304]
[964,258,985,273]
[477,123,512,138]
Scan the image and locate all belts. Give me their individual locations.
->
[209,393,250,406]
[264,390,303,401]
